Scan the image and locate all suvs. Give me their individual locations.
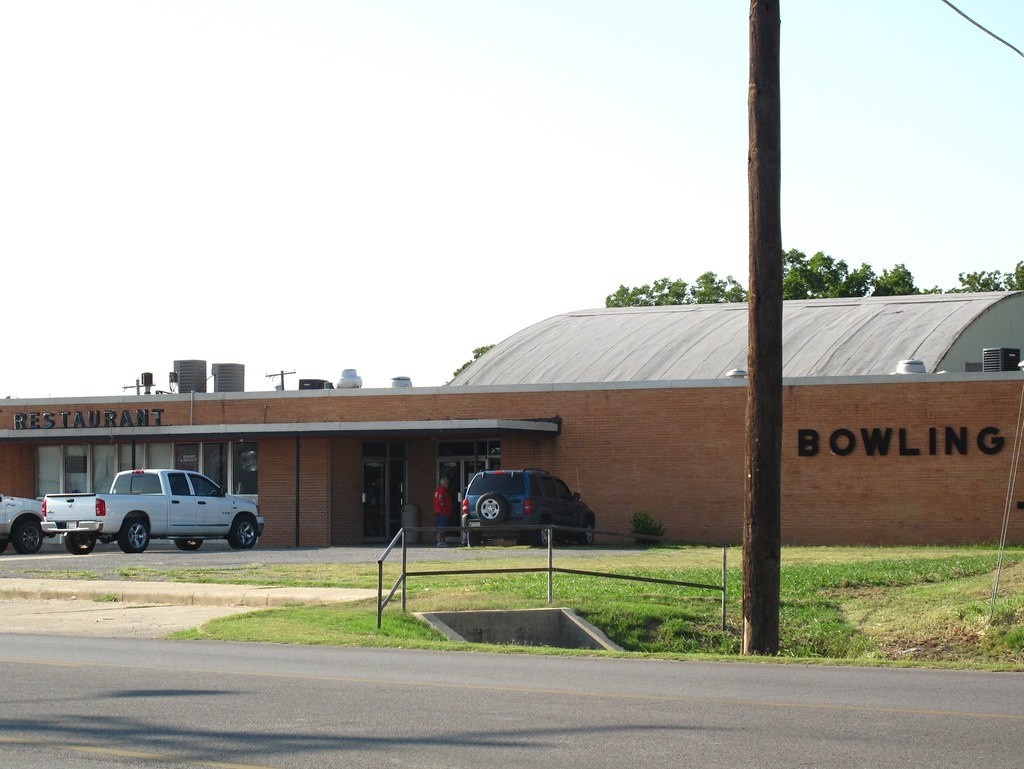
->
[0,493,57,555]
[458,467,596,549]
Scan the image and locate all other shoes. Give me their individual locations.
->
[437,543,449,548]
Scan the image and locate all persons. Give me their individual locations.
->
[434,477,453,547]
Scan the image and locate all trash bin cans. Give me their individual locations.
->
[401,503,420,544]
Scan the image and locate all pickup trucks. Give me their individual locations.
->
[40,469,265,551]
